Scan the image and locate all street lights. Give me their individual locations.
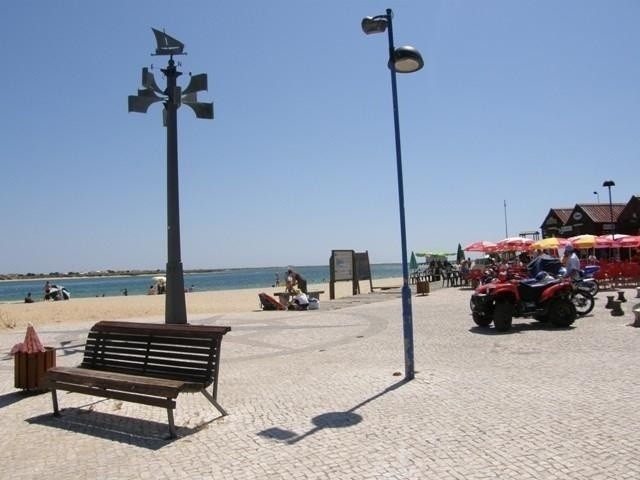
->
[361,8,424,379]
[604,181,615,240]
[593,191,600,204]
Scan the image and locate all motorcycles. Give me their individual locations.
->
[570,265,599,315]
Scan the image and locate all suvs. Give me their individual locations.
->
[470,270,575,331]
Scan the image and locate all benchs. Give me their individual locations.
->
[274,290,325,306]
[42,320,231,439]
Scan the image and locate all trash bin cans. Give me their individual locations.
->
[417,282,429,296]
[15,346,56,391]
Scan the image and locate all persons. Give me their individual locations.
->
[42,281,51,302]
[275,272,281,287]
[563,246,582,277]
[292,288,309,310]
[460,257,470,286]
[158,279,164,294]
[288,269,307,294]
[423,259,454,279]
[471,259,483,288]
[25,292,34,303]
[149,285,157,295]
[285,281,296,294]
[284,272,288,282]
[452,260,462,276]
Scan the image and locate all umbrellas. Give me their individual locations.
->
[415,248,457,261]
[49,284,71,300]
[456,243,466,264]
[409,251,419,277]
[460,232,640,265]
[152,276,166,283]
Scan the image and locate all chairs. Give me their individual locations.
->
[409,258,640,290]
